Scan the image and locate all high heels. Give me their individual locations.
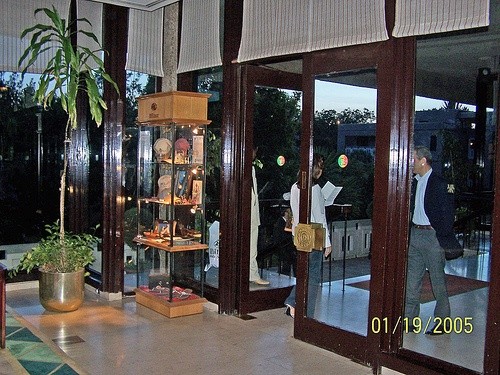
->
[285,304,294,318]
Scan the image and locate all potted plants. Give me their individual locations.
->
[9,4,120,312]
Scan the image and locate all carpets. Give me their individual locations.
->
[0,305,89,375]
[347,270,490,304]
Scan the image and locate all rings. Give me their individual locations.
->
[328,252,329,253]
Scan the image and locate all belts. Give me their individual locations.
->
[413,224,434,231]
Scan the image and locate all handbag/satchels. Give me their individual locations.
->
[283,208,292,231]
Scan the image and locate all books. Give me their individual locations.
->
[321,181,343,206]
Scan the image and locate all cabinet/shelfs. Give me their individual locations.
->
[133,91,212,318]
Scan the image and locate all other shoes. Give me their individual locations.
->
[253,278,270,285]
[403,326,416,332]
[426,324,453,335]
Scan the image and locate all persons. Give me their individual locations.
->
[403,147,464,336]
[249,144,270,285]
[284,153,332,318]
